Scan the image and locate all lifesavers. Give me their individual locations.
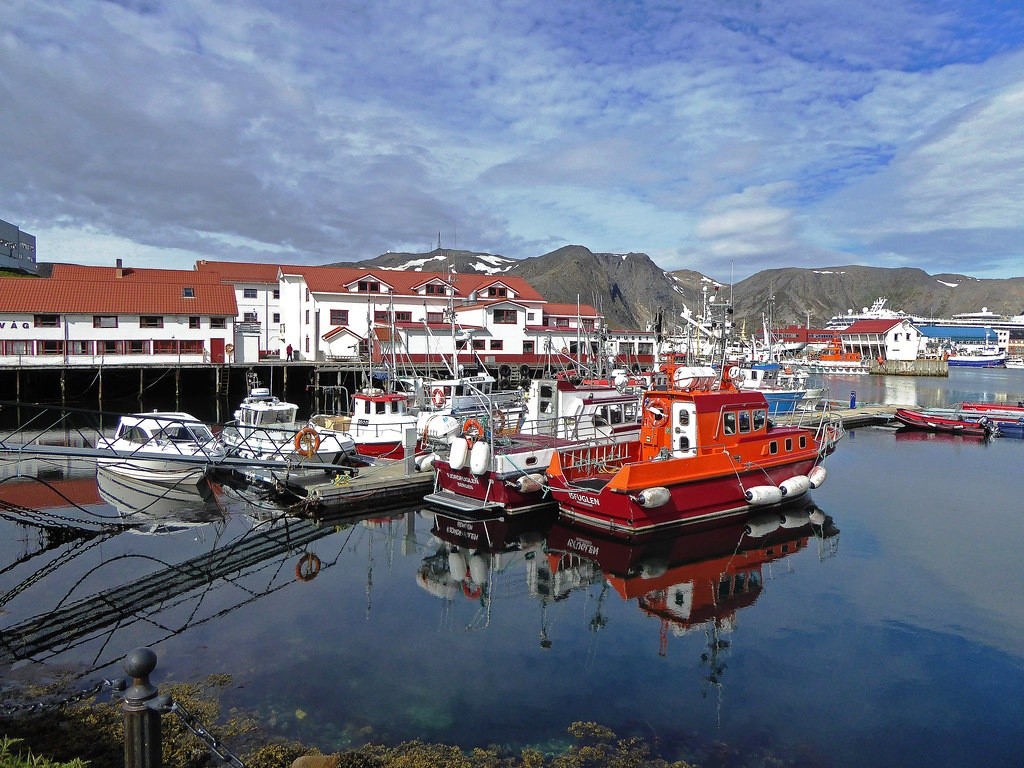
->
[432,388,444,406]
[645,399,670,428]
[484,408,505,434]
[294,553,322,581]
[462,419,483,449]
[296,427,321,456]
[225,345,234,352]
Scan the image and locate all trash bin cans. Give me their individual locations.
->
[293,349,300,361]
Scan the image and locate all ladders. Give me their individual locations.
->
[217,361,232,400]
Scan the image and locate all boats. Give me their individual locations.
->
[548,388,846,538]
[895,406,997,438]
[220,387,357,472]
[307,288,812,528]
[956,402,1024,431]
[97,466,840,734]
[952,351,1010,369]
[98,405,219,483]
